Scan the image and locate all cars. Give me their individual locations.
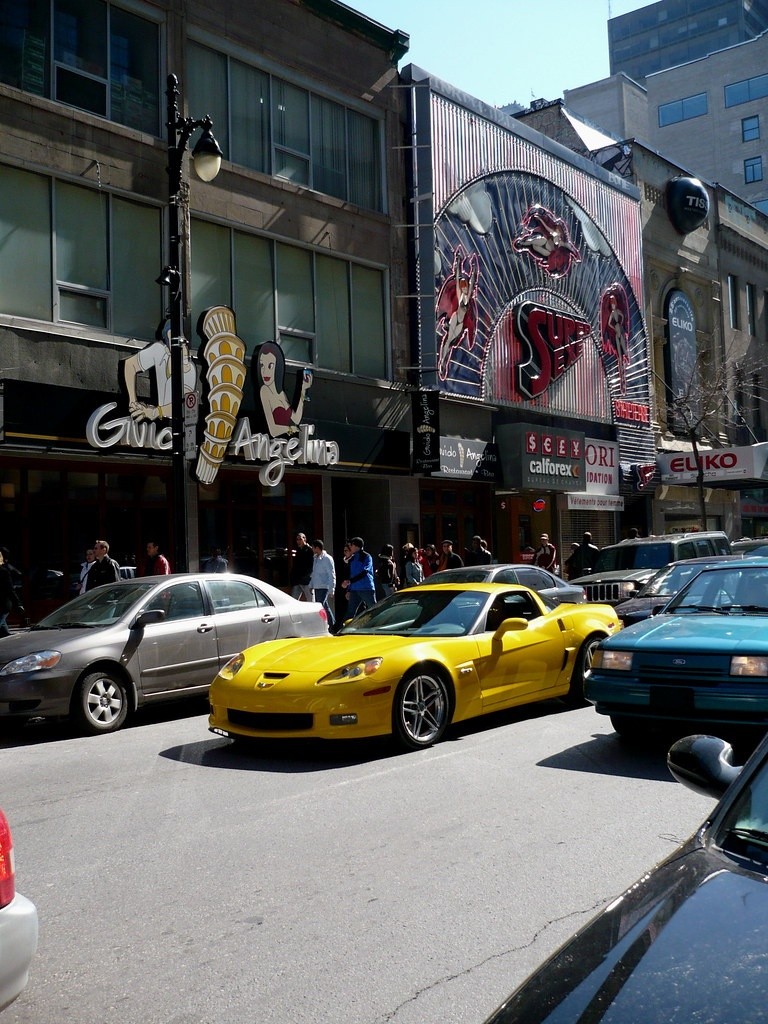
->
[1,573,332,740]
[612,553,763,628]
[730,536,768,558]
[485,714,768,1023]
[581,556,768,738]
[347,563,587,650]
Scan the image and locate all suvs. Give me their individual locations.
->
[571,530,736,606]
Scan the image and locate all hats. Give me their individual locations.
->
[440,540,453,546]
[540,534,549,540]
[345,537,364,548]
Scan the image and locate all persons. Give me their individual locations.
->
[77,533,492,620]
[564,528,601,586]
[0,548,24,639]
[533,534,556,581]
[619,528,638,571]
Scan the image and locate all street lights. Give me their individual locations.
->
[153,69,224,569]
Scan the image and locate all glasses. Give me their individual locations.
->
[443,545,450,547]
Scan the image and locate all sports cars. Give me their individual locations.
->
[210,582,625,753]
